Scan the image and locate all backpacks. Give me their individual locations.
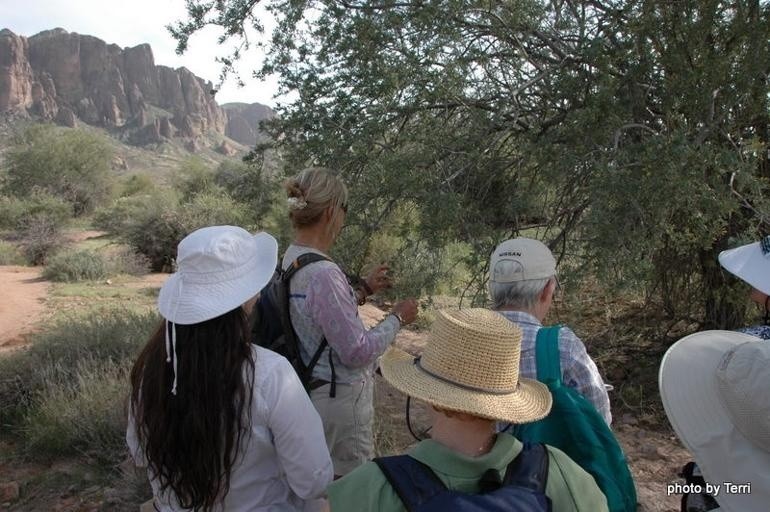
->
[372,442,553,512]
[517,328,643,512]
[251,252,333,402]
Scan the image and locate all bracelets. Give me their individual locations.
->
[391,310,404,323]
[363,279,374,296]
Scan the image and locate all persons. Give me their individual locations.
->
[717,230,768,337]
[326,308,613,512]
[485,236,613,439]
[125,225,336,512]
[280,164,418,478]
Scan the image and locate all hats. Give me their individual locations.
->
[158,224,278,325]
[489,237,556,282]
[374,302,558,427]
